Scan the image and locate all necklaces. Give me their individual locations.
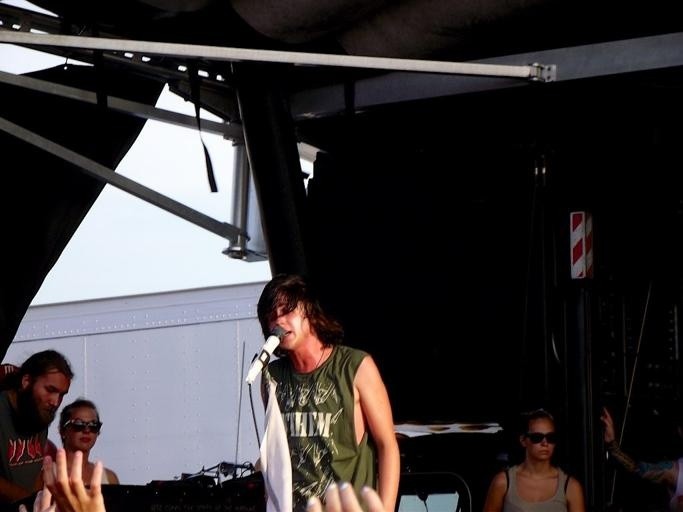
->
[285,343,331,375]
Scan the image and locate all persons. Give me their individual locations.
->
[255,271,401,511]
[40,447,108,512]
[41,398,121,485]
[596,404,682,511]
[306,480,385,512]
[0,347,75,511]
[482,409,583,511]
[15,483,57,512]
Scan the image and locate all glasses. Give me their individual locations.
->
[62,417,103,436]
[522,431,556,445]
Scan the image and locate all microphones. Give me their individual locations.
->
[245,327,286,385]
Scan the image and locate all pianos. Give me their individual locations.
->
[4,466,264,512]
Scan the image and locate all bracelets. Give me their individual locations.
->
[607,439,616,445]
